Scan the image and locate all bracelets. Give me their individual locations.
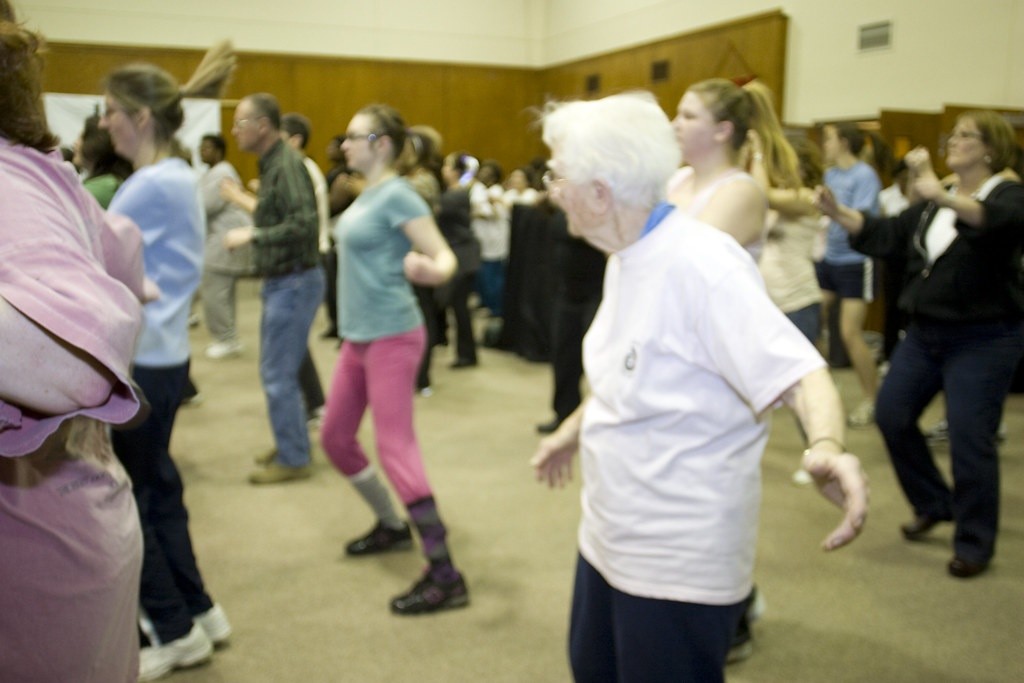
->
[807,436,845,453]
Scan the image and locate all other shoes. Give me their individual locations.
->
[450,350,477,369]
[744,588,763,618]
[900,504,954,541]
[389,571,472,613]
[928,420,1007,450]
[537,417,564,435]
[193,602,232,642]
[844,399,879,431]
[723,614,753,663]
[247,458,315,485]
[186,313,198,328]
[948,549,992,578]
[137,620,214,683]
[204,338,245,361]
[254,444,281,464]
[307,408,324,425]
[346,520,413,556]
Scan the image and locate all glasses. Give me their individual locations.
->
[339,131,378,142]
[234,116,266,133]
[101,102,123,119]
[949,127,985,143]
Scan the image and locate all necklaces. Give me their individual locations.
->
[954,176,990,197]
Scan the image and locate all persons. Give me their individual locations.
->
[320,104,469,614]
[98,38,237,682]
[74,89,504,484]
[0,0,161,683]
[528,92,868,683]
[504,77,1024,577]
